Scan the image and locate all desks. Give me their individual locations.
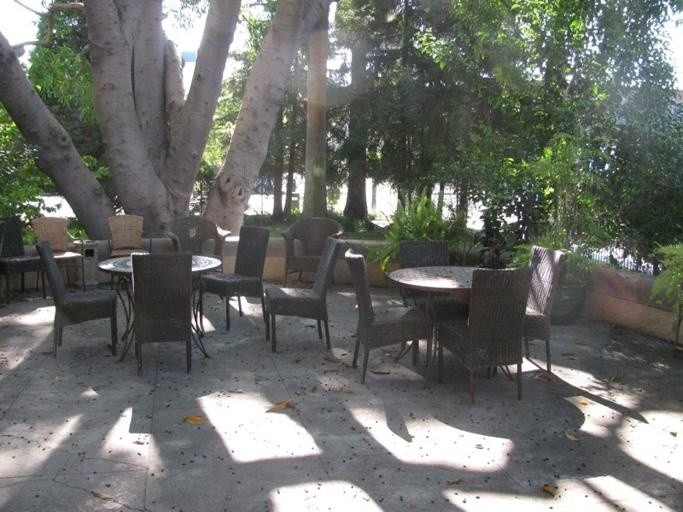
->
[97,254,224,363]
[387,266,489,363]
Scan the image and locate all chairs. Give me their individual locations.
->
[37,241,119,359]
[281,215,345,288]
[201,225,270,330]
[487,245,566,378]
[399,239,470,319]
[164,216,232,298]
[132,253,194,376]
[343,249,433,385]
[438,265,536,405]
[1,214,46,305]
[265,235,341,353]
[31,217,84,297]
[107,214,146,287]
